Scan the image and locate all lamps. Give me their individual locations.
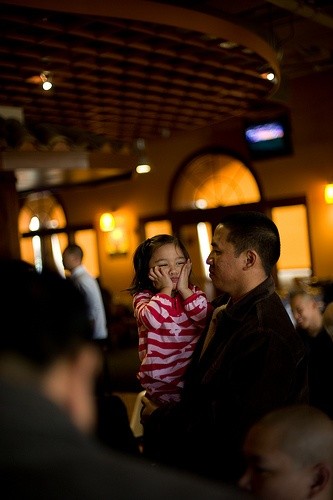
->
[40,73,52,90]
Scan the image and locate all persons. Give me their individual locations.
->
[289,290,333,339]
[121,234,214,406]
[0,254,230,500]
[238,405,333,499]
[63,245,108,346]
[141,211,299,456]
